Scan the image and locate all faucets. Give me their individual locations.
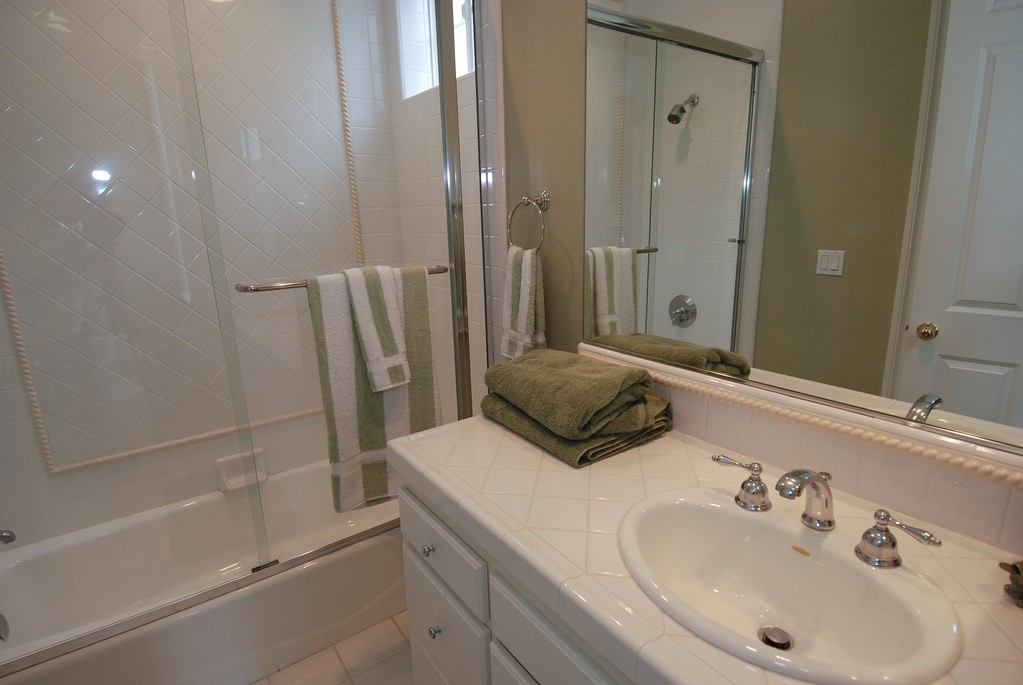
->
[905,393,943,424]
[775,468,837,531]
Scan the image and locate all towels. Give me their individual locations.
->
[480,348,674,468]
[591,332,751,382]
[305,264,443,514]
[584,245,638,340]
[500,244,548,361]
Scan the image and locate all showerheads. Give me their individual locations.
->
[666,93,699,125]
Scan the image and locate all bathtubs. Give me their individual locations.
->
[0,454,409,685]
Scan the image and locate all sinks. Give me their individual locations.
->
[616,487,965,685]
